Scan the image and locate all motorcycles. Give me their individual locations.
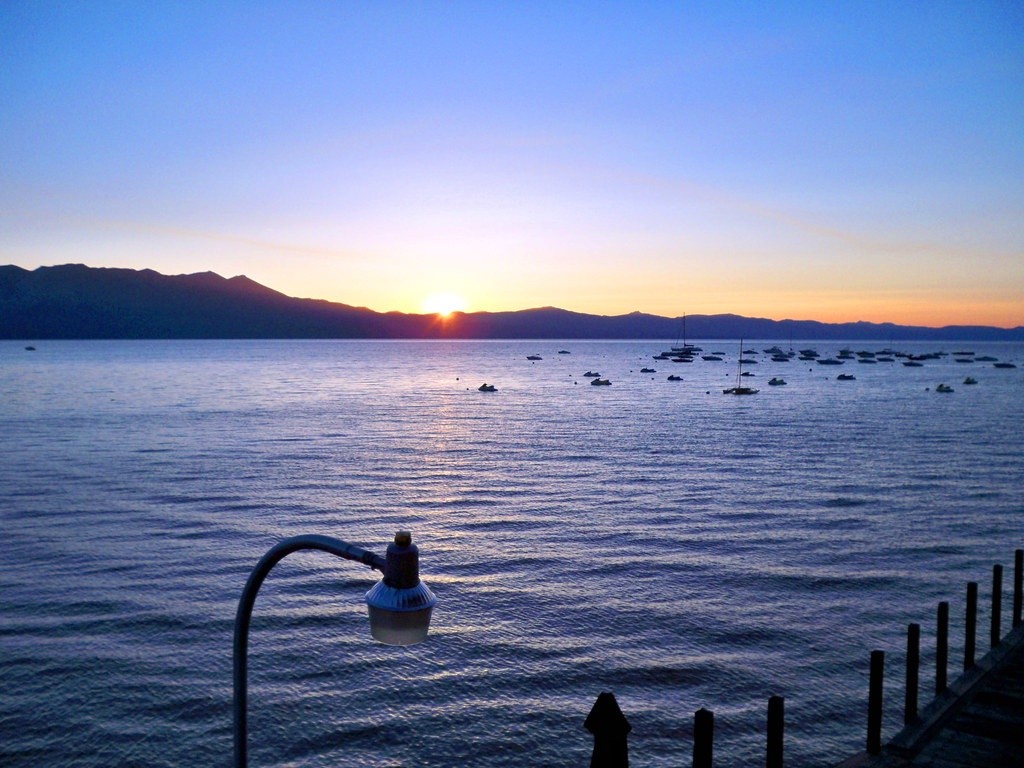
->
[962,376,979,386]
[768,377,787,386]
[590,378,612,386]
[640,367,657,373]
[837,373,856,380]
[478,383,498,392]
[936,384,954,393]
[740,372,755,377]
[583,371,601,377]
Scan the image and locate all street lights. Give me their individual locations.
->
[231,528,437,767]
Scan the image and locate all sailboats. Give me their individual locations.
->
[723,337,760,395]
[671,312,703,351]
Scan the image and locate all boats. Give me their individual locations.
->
[558,350,571,354]
[743,349,759,355]
[526,355,543,360]
[738,358,758,364]
[667,375,684,380]
[763,346,1017,369]
[652,354,723,363]
